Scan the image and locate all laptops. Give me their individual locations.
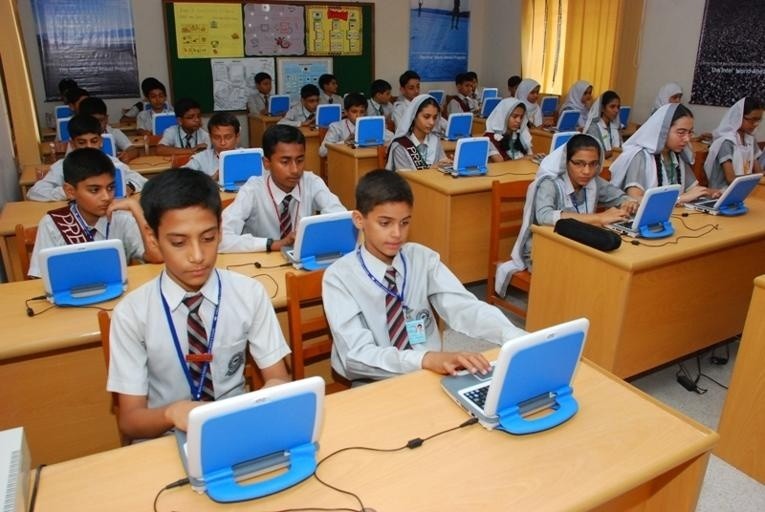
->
[438,137,489,178]
[175,376,325,503]
[286,210,359,272]
[264,96,290,117]
[616,106,633,129]
[39,239,128,307]
[55,101,182,200]
[428,90,445,105]
[479,98,502,118]
[217,148,265,193]
[440,317,590,435]
[684,173,763,216]
[479,88,499,104]
[531,131,580,165]
[544,110,581,133]
[603,184,681,239]
[440,112,473,141]
[308,104,341,129]
[341,91,366,112]
[346,116,386,149]
[539,95,558,116]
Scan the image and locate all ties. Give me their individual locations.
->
[384,267,413,351]
[181,294,215,401]
[280,195,292,240]
[184,134,193,148]
[264,96,268,105]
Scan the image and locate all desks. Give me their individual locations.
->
[3,107,763,512]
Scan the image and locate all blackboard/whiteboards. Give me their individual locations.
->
[160,0,376,118]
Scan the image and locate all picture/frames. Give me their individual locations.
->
[276,56,335,106]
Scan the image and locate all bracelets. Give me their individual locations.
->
[267,238,273,251]
[677,197,681,205]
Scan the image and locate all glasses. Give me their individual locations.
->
[570,159,599,168]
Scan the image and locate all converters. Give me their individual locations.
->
[711,357,726,364]
[678,376,696,392]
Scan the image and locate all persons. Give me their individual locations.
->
[605,103,725,208]
[482,76,556,163]
[216,124,346,254]
[321,168,533,388]
[386,94,453,171]
[26,78,150,201]
[104,167,292,446]
[278,74,396,155]
[27,147,163,279]
[249,73,273,118]
[391,70,447,134]
[704,96,765,189]
[521,134,638,272]
[558,80,624,157]
[447,73,483,116]
[120,77,270,180]
[645,82,713,144]
